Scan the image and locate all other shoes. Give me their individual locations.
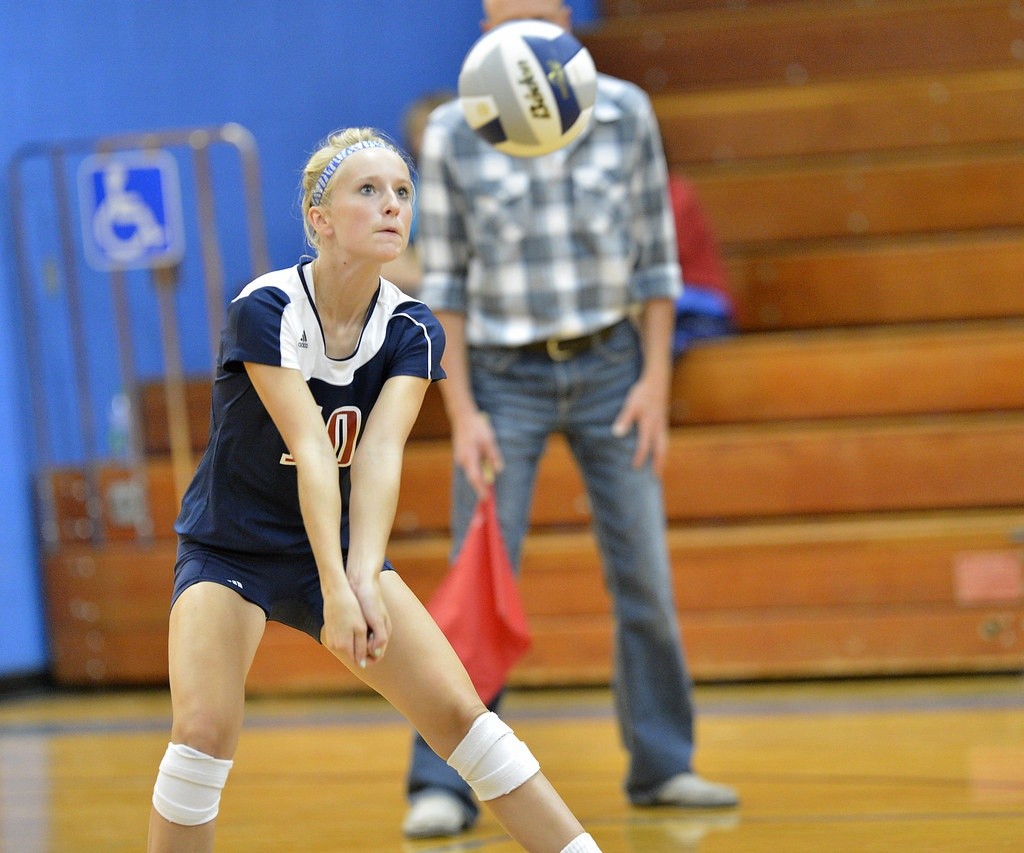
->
[650,771,736,806]
[403,786,464,842]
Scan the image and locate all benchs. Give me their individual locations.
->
[27,0,1023,703]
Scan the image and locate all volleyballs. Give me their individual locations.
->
[455,15,600,162]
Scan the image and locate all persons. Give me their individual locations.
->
[137,124,600,852]
[395,0,742,839]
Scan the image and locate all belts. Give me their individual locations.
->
[521,322,618,363]
